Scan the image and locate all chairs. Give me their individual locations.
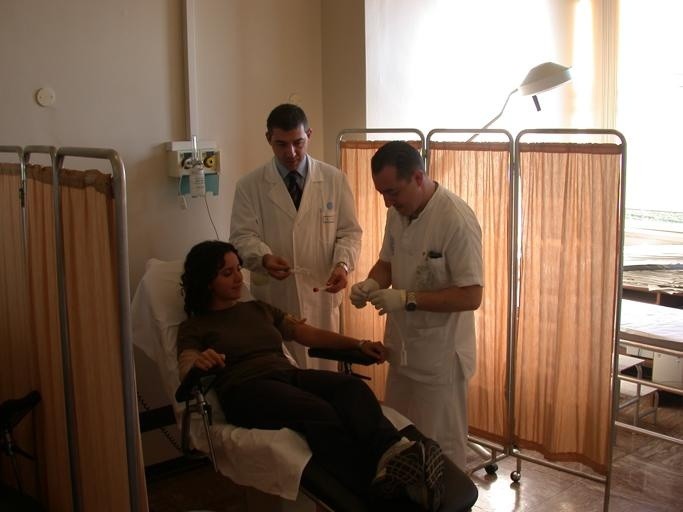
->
[130,255,479,512]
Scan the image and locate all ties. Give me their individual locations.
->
[286,171,302,211]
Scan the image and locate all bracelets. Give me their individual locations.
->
[358,340,368,350]
[336,263,348,277]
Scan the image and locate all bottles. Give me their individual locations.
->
[189,150,207,199]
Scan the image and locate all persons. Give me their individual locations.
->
[175,239,478,511]
[228,104,363,374]
[349,142,485,474]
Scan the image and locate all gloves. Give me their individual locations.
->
[368,289,407,316]
[350,279,380,308]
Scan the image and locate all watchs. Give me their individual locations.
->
[406,290,417,312]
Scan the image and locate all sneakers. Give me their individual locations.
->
[371,441,423,494]
[407,441,447,511]
